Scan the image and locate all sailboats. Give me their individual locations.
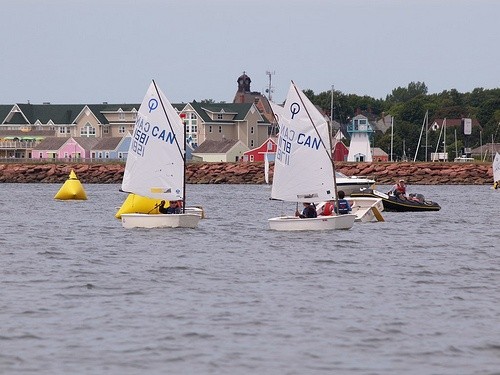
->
[266,79,359,231]
[119,78,207,228]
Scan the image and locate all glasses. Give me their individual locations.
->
[170,202,177,204]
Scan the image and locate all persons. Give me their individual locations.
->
[387,179,407,201]
[296,203,317,219]
[159,200,182,214]
[494,181,498,189]
[323,190,352,215]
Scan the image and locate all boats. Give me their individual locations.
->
[333,166,377,196]
[372,189,442,213]
[339,193,384,224]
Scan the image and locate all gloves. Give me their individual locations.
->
[160,201,165,205]
